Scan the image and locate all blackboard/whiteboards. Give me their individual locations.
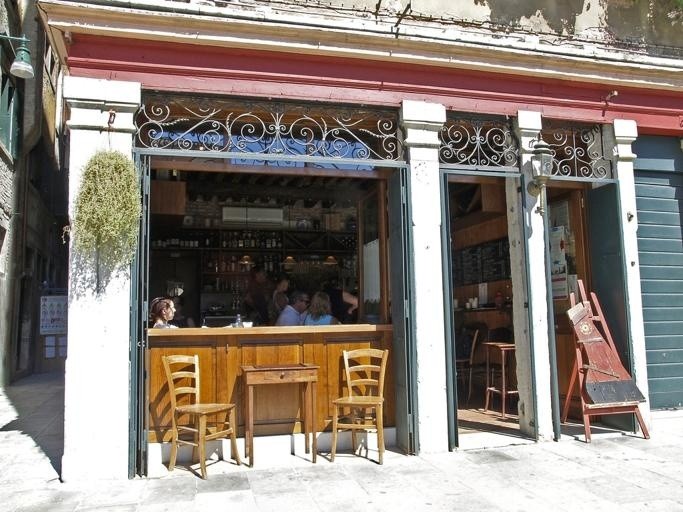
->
[452,236,511,288]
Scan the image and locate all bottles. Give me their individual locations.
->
[234,314,245,328]
[463,296,478,309]
[151,229,286,312]
[495,284,512,308]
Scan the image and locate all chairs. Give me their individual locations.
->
[457,329,511,411]
[162,348,389,478]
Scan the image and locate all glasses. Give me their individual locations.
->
[299,299,311,305]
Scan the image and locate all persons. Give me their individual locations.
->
[244,264,270,327]
[165,295,196,329]
[273,289,310,326]
[266,271,290,327]
[302,291,343,324]
[149,295,180,330]
[306,270,358,324]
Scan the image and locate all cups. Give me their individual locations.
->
[454,298,458,309]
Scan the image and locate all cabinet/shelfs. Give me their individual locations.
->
[149,211,361,327]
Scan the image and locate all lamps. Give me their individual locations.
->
[528,132,553,215]
[0,32,35,81]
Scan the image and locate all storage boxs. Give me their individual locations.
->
[150,180,185,216]
